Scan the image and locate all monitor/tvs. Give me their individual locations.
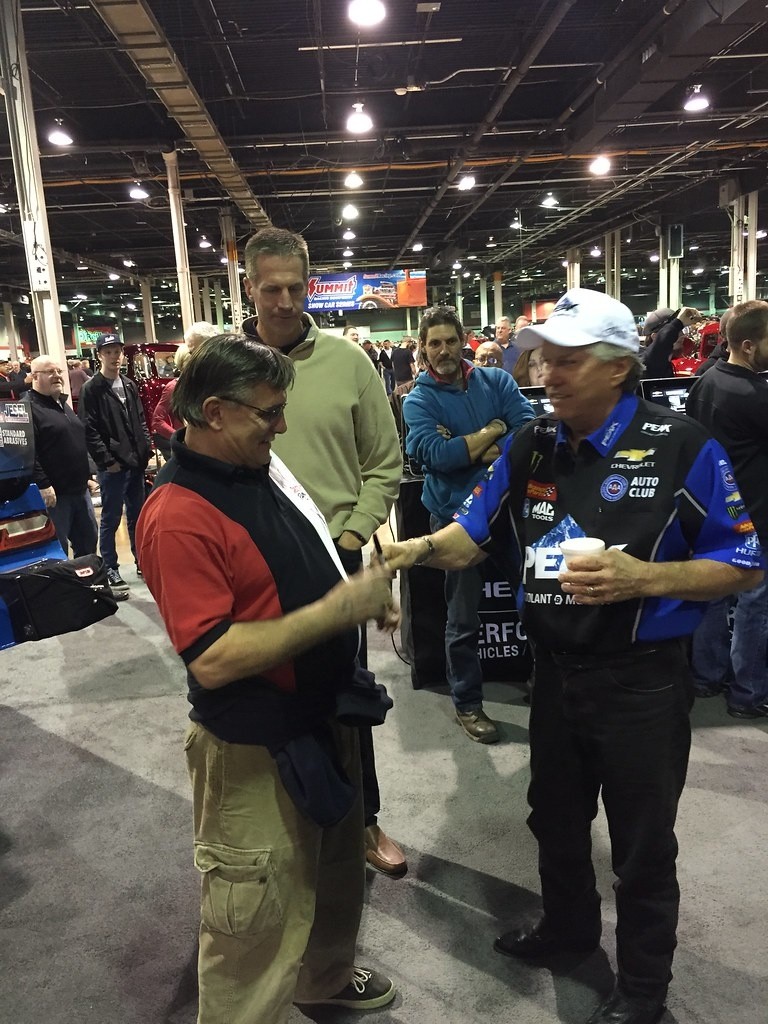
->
[641,376,701,417]
[518,386,555,416]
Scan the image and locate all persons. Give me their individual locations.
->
[369,287,766,1024]
[130,333,402,1024]
[0,305,768,744]
[238,227,408,877]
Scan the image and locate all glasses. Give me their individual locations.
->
[219,396,288,422]
[34,369,63,376]
[476,357,504,365]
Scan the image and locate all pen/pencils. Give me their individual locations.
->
[372,533,384,564]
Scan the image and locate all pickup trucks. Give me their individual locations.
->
[0,343,181,443]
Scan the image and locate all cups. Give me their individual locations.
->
[560,537,606,570]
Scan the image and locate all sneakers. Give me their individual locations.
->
[107,567,129,590]
[137,569,142,578]
[292,965,395,1010]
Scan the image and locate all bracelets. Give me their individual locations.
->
[406,536,436,566]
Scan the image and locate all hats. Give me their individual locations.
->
[0,360,8,364]
[644,309,681,336]
[96,334,125,351]
[517,288,640,352]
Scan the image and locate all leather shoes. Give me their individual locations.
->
[585,985,667,1024]
[364,825,408,880]
[494,926,601,960]
[454,706,498,743]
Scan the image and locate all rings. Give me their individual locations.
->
[587,584,594,597]
[445,428,451,435]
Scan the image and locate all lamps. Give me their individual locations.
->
[683,86,709,109]
[509,219,520,228]
[486,238,497,247]
[199,236,212,247]
[48,118,74,146]
[453,260,461,268]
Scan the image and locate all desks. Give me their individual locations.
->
[394,471,534,691]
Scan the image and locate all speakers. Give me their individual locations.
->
[668,223,684,258]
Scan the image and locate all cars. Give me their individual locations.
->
[671,319,724,379]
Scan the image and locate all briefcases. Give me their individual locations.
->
[0,553,119,645]
